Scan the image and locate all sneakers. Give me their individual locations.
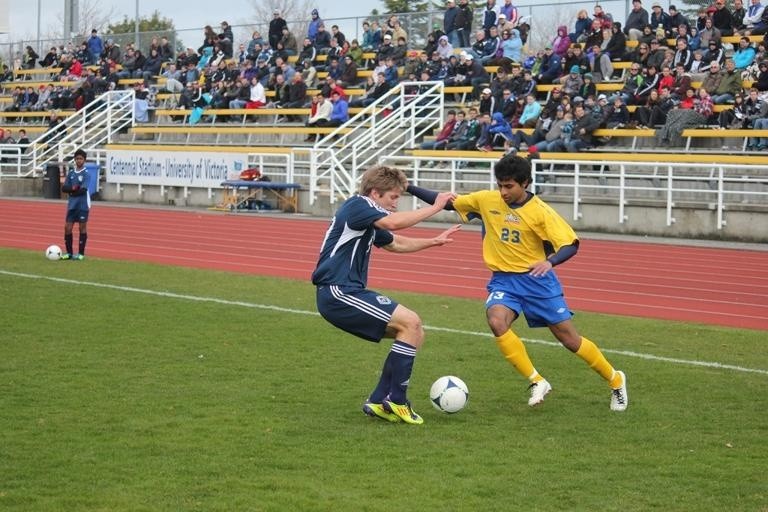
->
[363,392,424,425]
[525,378,552,408]
[609,370,628,412]
[60,252,85,261]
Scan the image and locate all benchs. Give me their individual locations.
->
[0,0,767,166]
[222,180,300,214]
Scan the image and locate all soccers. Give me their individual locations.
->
[46,245,62,260]
[430,376,469,414]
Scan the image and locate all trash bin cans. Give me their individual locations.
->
[42,164,61,199]
[82,163,101,197]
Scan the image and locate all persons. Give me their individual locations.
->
[396,156,628,412]
[386,1,767,171]
[0,29,173,168]
[63,150,92,260]
[311,167,461,425]
[157,9,408,144]
